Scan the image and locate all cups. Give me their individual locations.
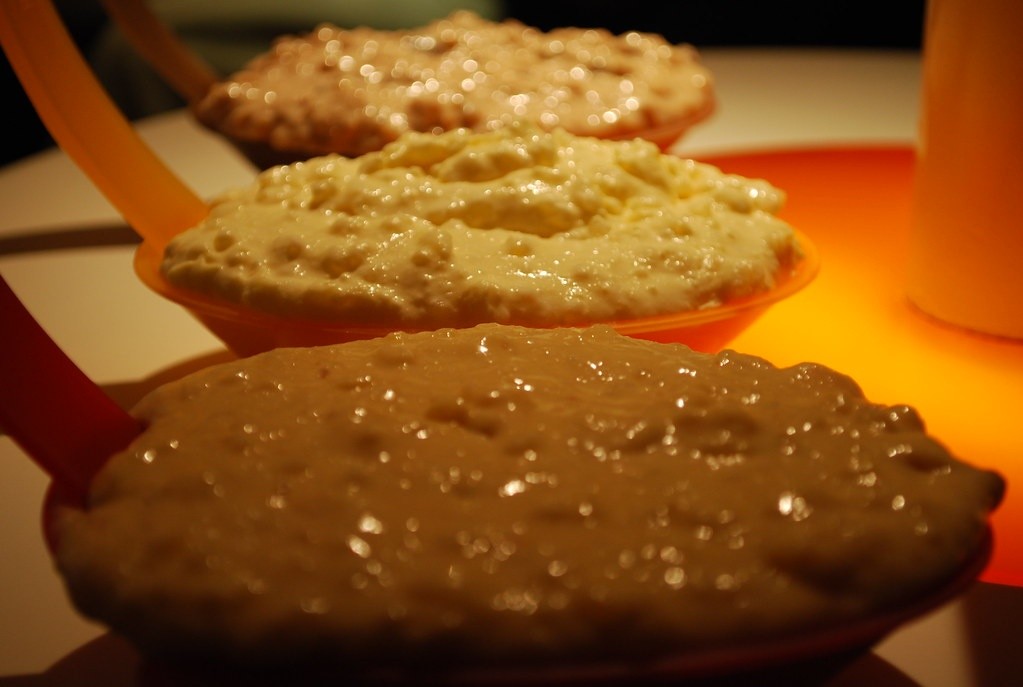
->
[900,0,1022,346]
